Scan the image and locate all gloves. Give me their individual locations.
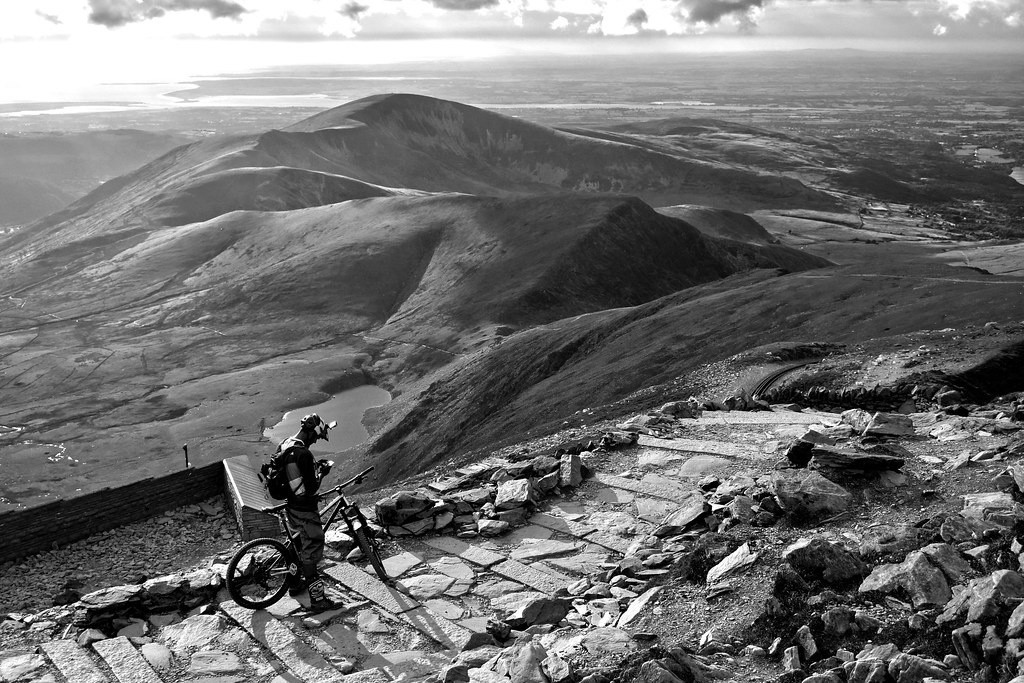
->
[316,459,331,476]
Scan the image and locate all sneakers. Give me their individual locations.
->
[289,574,307,596]
[310,595,344,613]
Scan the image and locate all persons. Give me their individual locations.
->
[275,413,345,611]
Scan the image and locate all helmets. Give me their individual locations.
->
[300,413,329,441]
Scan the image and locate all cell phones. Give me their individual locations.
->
[325,460,334,467]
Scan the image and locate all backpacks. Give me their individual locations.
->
[256,437,304,500]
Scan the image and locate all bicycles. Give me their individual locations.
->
[225,464,388,611]
